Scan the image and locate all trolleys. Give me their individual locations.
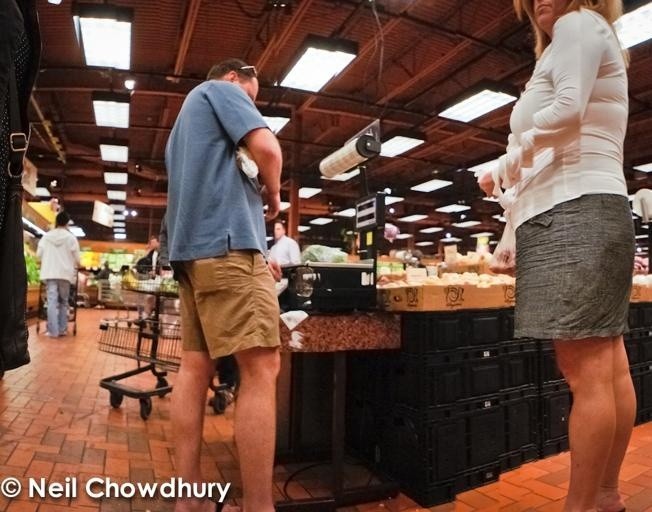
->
[36,267,83,336]
[97,264,229,421]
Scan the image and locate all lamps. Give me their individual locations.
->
[91,89,132,130]
[436,78,520,124]
[271,34,360,96]
[69,1,132,73]
[256,106,292,135]
[97,139,129,239]
[263,166,496,247]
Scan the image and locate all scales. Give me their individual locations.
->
[279,193,386,311]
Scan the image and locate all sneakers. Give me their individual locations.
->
[44,331,67,338]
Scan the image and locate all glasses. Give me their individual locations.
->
[240,65,258,77]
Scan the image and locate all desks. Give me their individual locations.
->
[275,314,403,511]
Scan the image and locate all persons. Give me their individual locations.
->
[165,58,284,511]
[477,0,638,511]
[34,210,79,339]
[402,251,429,275]
[77,235,167,325]
[0,1,43,372]
[266,221,304,267]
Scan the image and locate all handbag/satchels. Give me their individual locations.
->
[0,177,30,370]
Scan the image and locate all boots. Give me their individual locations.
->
[234,145,259,179]
